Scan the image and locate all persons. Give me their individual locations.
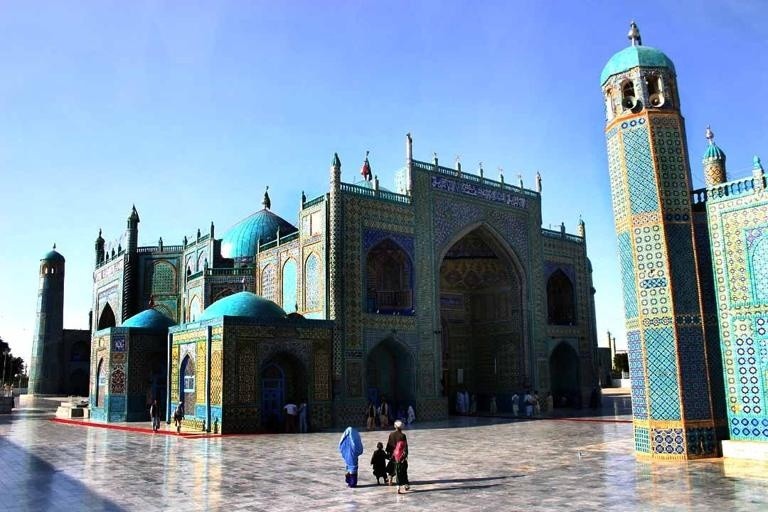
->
[488,391,498,416]
[174,401,184,434]
[282,398,298,433]
[340,426,363,487]
[392,440,410,493]
[455,388,477,415]
[365,400,376,430]
[294,398,308,433]
[407,405,416,424]
[371,442,388,485]
[377,396,390,428]
[589,386,600,411]
[385,419,408,486]
[150,399,161,433]
[510,390,554,418]
